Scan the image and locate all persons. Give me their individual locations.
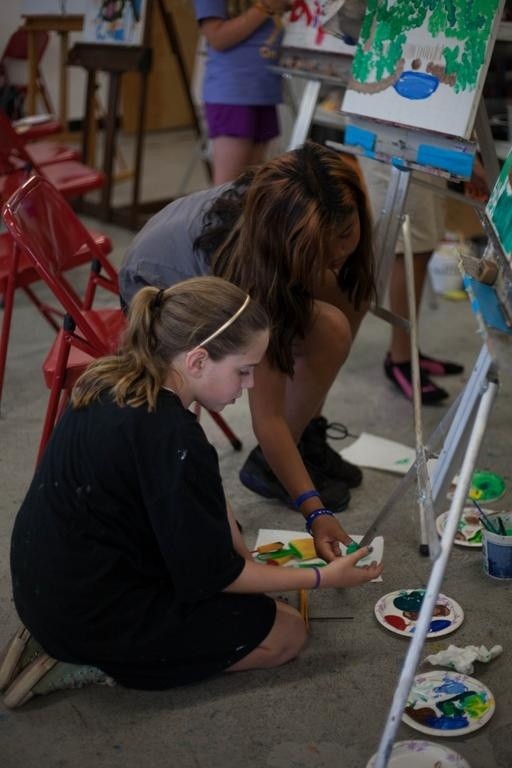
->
[188,0,299,188]
[2,277,386,711]
[114,143,367,560]
[353,153,492,408]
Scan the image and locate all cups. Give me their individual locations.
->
[481,511,511,582]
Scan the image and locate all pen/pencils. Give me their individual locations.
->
[497,302,512,328]
[300,589,310,631]
[309,618,354,620]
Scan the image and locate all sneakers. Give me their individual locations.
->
[383,351,449,403]
[239,442,352,513]
[409,349,464,376]
[297,414,363,490]
[3,650,114,708]
[0,622,43,691]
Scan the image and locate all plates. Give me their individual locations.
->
[436,506,489,548]
[444,470,506,503]
[403,670,494,735]
[367,742,469,768]
[374,589,463,639]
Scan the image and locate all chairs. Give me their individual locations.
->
[3,175,243,475]
[0,87,125,389]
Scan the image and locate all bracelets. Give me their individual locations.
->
[301,507,336,535]
[309,565,324,590]
[288,489,324,511]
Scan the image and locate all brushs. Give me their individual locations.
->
[250,543,282,554]
[258,539,317,561]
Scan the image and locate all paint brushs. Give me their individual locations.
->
[473,500,507,536]
